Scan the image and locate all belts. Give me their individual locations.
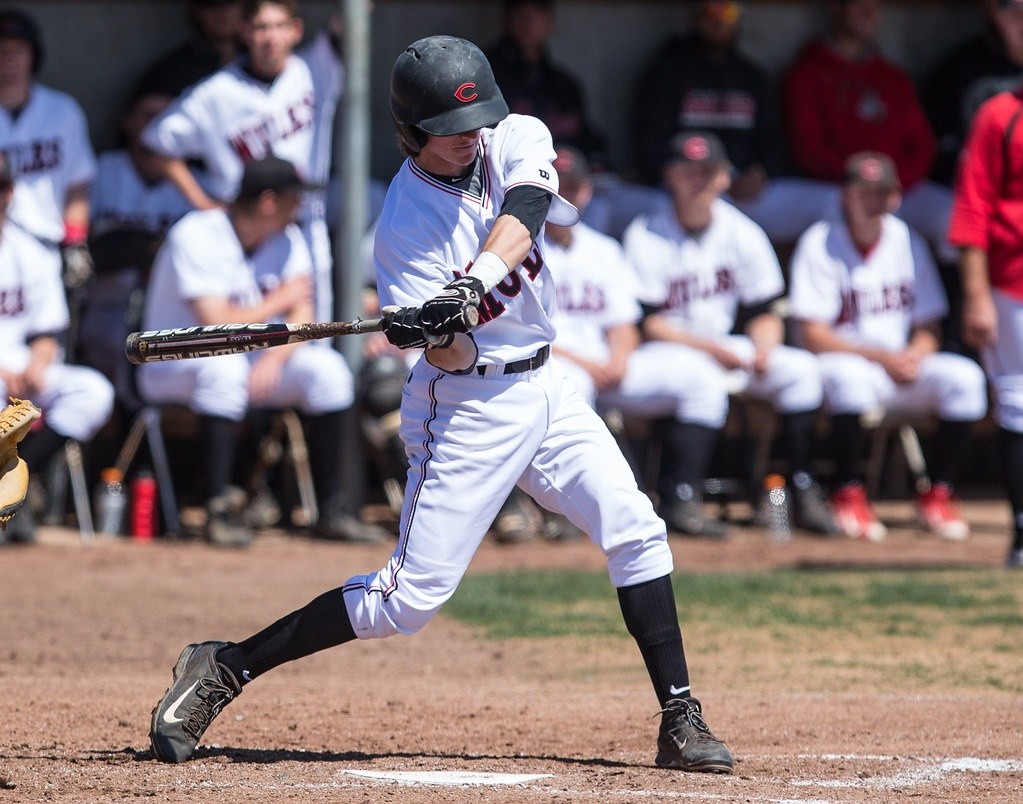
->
[476,344,552,376]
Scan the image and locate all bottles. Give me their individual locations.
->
[761,473,792,542]
[128,462,161,545]
[95,467,126,533]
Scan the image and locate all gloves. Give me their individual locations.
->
[66,242,96,288]
[423,276,485,334]
[383,308,455,349]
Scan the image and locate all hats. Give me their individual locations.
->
[844,155,899,189]
[389,37,510,153]
[662,128,737,179]
[242,158,333,199]
[555,147,589,190]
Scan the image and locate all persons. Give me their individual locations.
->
[0,8,116,546]
[916,0,1023,190]
[623,0,843,246]
[73,0,386,552]
[786,152,990,543]
[947,83,1022,573]
[621,132,843,539]
[775,0,966,266]
[147,35,734,774]
[326,0,729,547]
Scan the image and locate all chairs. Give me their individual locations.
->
[35,281,186,537]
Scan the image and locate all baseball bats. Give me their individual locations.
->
[123,301,482,365]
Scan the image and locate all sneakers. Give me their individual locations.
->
[0,496,397,551]
[656,697,732,774]
[497,474,971,544]
[150,641,244,763]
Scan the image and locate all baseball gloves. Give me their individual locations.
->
[0,398,43,523]
